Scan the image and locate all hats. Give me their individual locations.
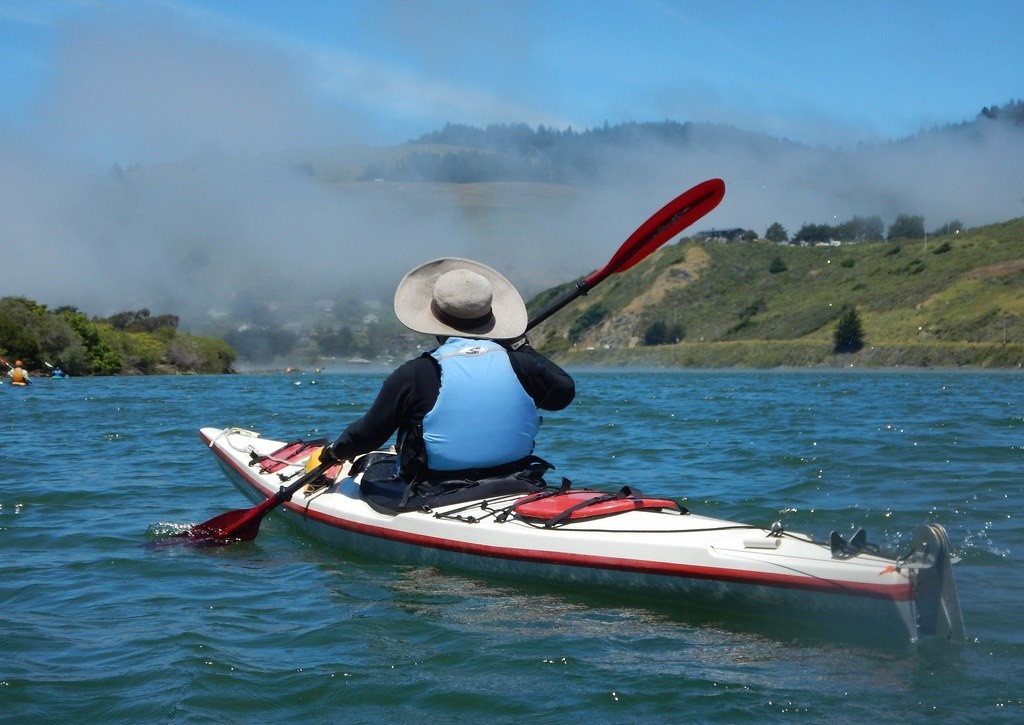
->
[393,256,528,340]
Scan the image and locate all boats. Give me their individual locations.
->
[51,374,70,379]
[10,381,26,387]
[199,425,969,643]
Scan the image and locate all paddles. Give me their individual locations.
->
[190,177,727,540]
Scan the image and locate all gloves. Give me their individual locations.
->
[318,441,344,467]
[501,333,531,351]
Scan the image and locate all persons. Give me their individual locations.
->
[51,366,63,375]
[318,256,576,497]
[7,360,32,383]
